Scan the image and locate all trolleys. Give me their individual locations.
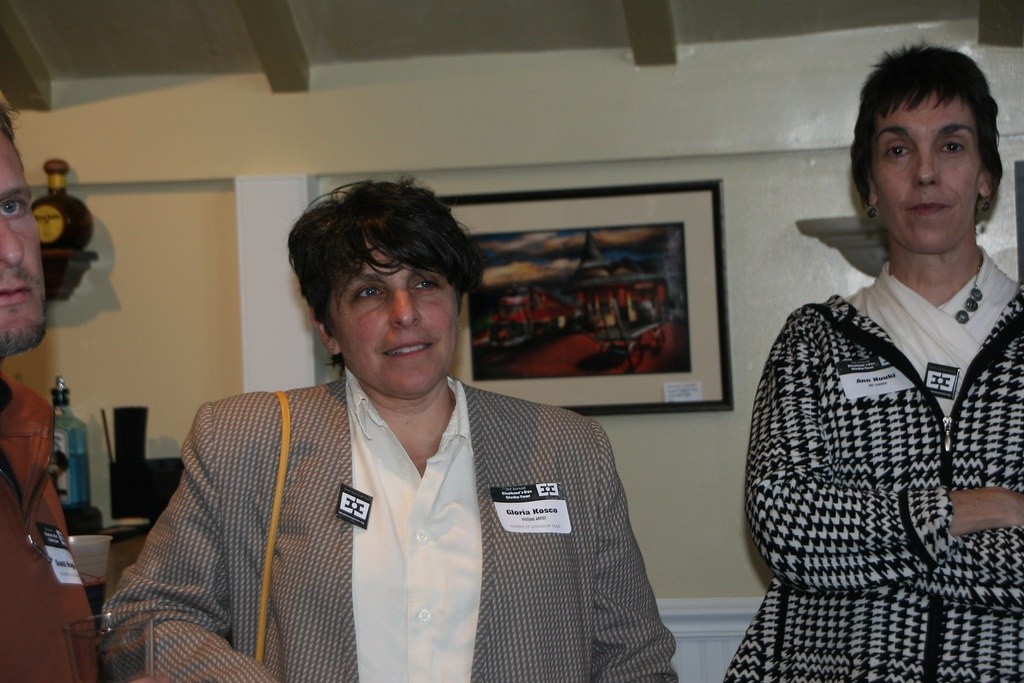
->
[579,273,668,367]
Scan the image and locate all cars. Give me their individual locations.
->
[490,293,571,348]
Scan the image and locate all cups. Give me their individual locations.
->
[66,534,113,621]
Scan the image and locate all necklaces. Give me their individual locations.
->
[955,255,983,324]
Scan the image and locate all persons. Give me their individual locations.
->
[100,180,680,683]
[721,46,1024,683]
[0,104,100,682]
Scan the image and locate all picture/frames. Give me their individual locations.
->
[440,179,734,415]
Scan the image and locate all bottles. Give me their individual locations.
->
[30,160,93,250]
[49,375,89,506]
